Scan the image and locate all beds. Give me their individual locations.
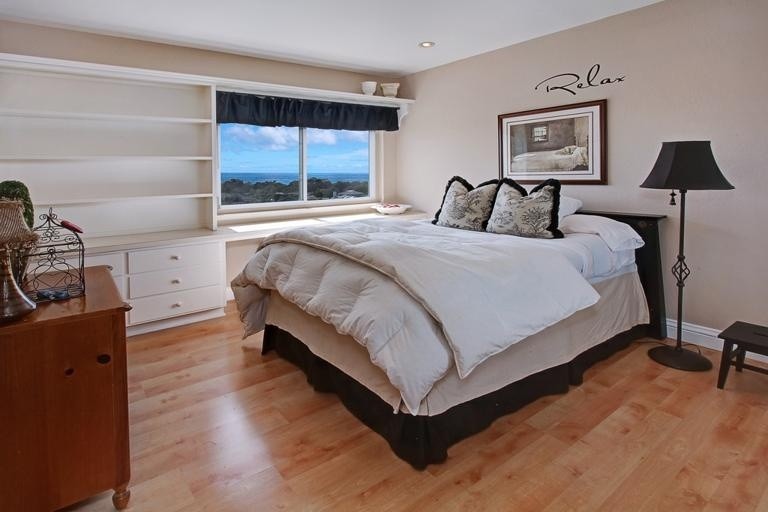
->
[261,206,669,471]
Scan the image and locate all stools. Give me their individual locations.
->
[714,319,766,388]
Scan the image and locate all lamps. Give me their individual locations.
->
[641,139,735,373]
[1,194,36,324]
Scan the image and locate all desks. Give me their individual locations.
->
[0,264,134,511]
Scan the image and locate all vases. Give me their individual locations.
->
[361,80,402,98]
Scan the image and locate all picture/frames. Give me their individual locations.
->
[497,98,608,187]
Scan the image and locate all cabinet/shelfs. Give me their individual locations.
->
[0,53,220,231]
[26,235,223,327]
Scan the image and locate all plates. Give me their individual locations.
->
[368,202,413,215]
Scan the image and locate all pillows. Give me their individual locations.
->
[432,178,584,238]
